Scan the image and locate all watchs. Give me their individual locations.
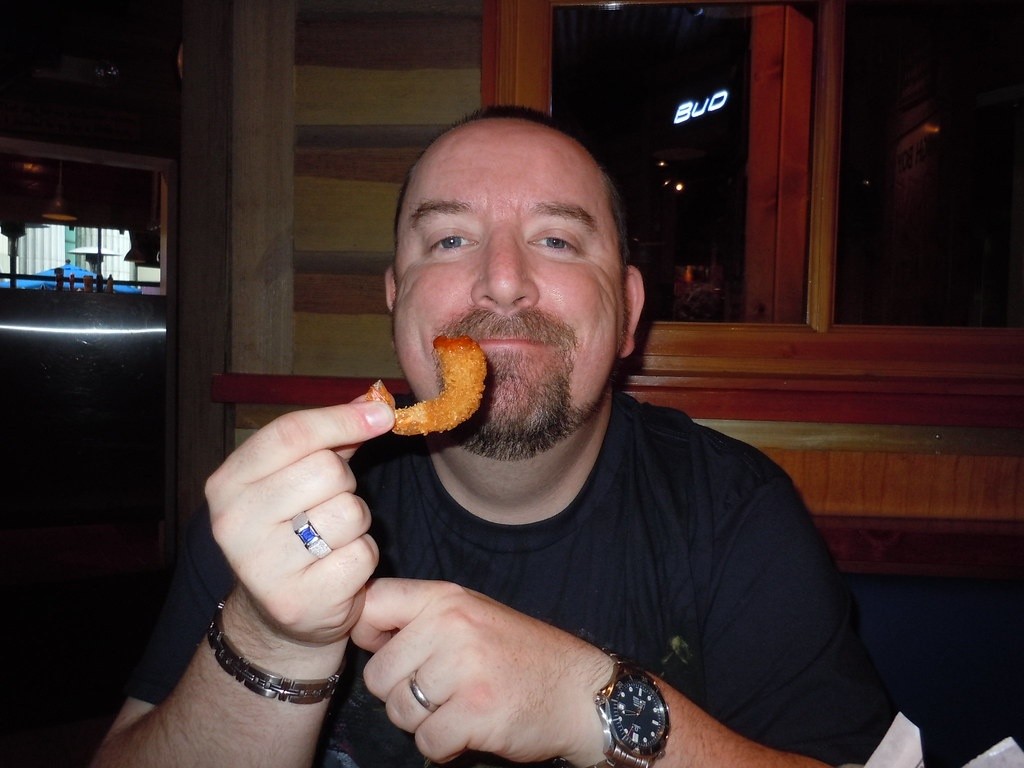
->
[592,647,672,768]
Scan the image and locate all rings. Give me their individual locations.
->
[291,511,333,560]
[408,672,437,713]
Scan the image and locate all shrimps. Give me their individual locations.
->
[367,336,489,437]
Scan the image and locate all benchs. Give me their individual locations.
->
[811,516,1024,768]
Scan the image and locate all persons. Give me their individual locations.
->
[90,105,1024,768]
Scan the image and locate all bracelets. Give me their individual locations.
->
[209,605,344,702]
